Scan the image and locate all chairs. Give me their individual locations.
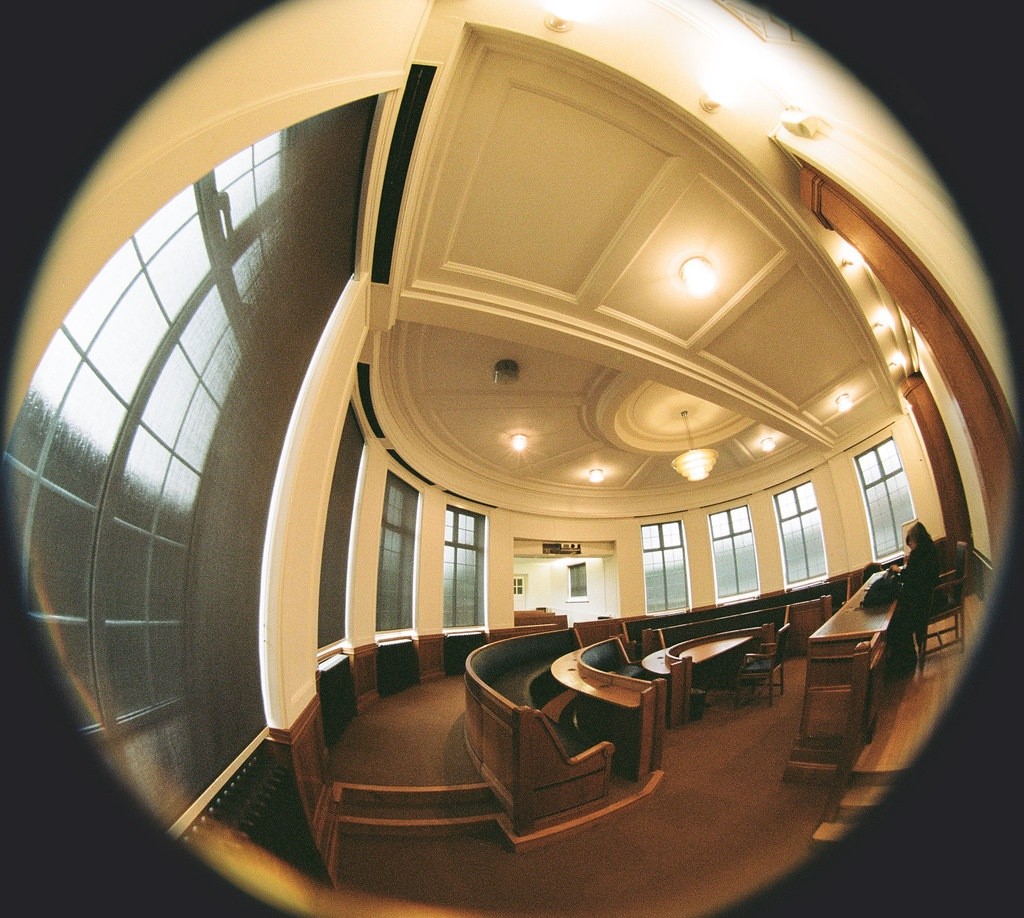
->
[919,541,969,670]
[861,562,884,585]
[736,623,792,707]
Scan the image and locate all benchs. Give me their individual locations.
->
[625,575,851,658]
[464,627,616,834]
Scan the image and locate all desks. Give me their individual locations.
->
[641,622,775,729]
[799,566,905,747]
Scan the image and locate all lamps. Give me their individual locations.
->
[511,434,529,451]
[836,393,852,412]
[590,469,604,483]
[761,437,775,452]
[681,256,720,295]
[671,411,719,482]
[842,253,861,269]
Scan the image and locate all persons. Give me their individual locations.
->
[887,523,941,671]
